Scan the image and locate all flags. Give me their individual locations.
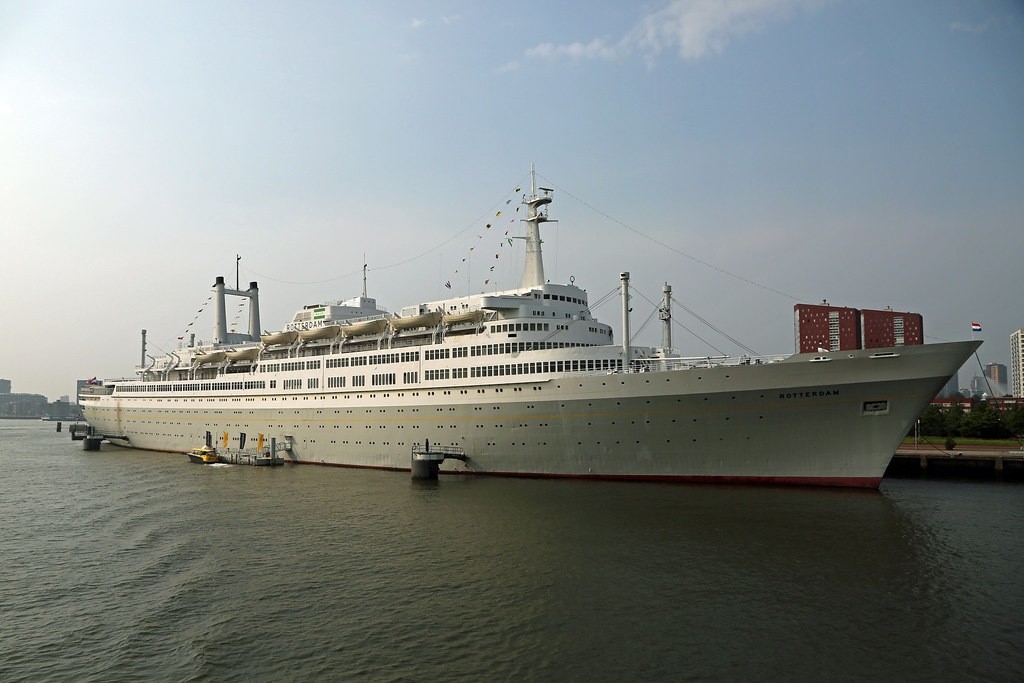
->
[445,189,522,289]
[89,377,96,383]
[230,298,247,332]
[972,323,981,331]
[177,296,212,339]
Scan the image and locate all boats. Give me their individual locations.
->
[69,159,984,491]
[186,445,219,464]
